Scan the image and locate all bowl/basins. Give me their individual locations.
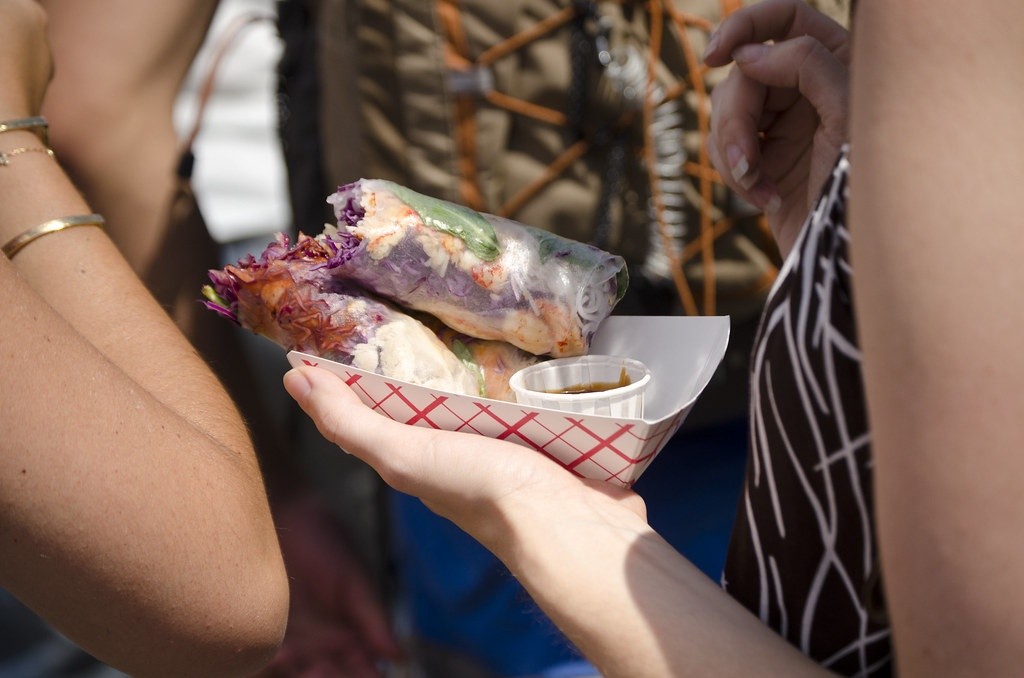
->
[509,354,651,418]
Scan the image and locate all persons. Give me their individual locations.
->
[283,1,1024,678]
[0,1,292,676]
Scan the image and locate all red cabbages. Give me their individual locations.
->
[205,184,616,367]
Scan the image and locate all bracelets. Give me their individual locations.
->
[0,117,105,261]
